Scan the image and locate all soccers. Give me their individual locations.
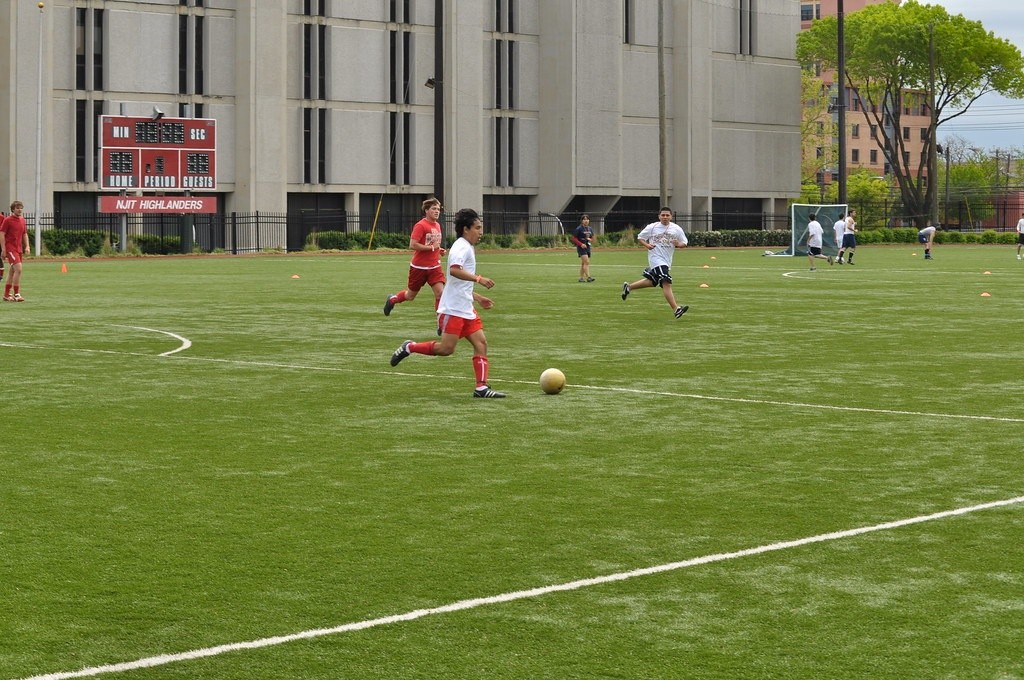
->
[540,368,566,394]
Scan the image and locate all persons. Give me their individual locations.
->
[807,214,831,273]
[572,215,596,282]
[384,196,446,319]
[390,209,506,398]
[895,218,903,227]
[839,209,858,265]
[0,200,30,302]
[1016,213,1024,260]
[620,207,689,319]
[833,213,846,263]
[918,225,943,260]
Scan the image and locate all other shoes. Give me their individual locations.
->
[1017,255,1021,260]
[838,260,844,264]
[810,267,816,272]
[828,256,834,265]
[924,255,934,259]
[847,260,855,264]
[835,259,844,263]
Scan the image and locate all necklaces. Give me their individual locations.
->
[582,225,589,237]
[651,222,670,243]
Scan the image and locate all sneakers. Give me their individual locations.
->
[674,304,689,319]
[621,282,629,300]
[473,388,506,398]
[579,279,585,282]
[3,295,14,301]
[384,293,395,316]
[13,294,24,301]
[437,327,441,336]
[390,339,412,367]
[587,277,595,282]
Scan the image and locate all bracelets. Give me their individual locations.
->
[476,275,481,283]
[430,245,434,252]
